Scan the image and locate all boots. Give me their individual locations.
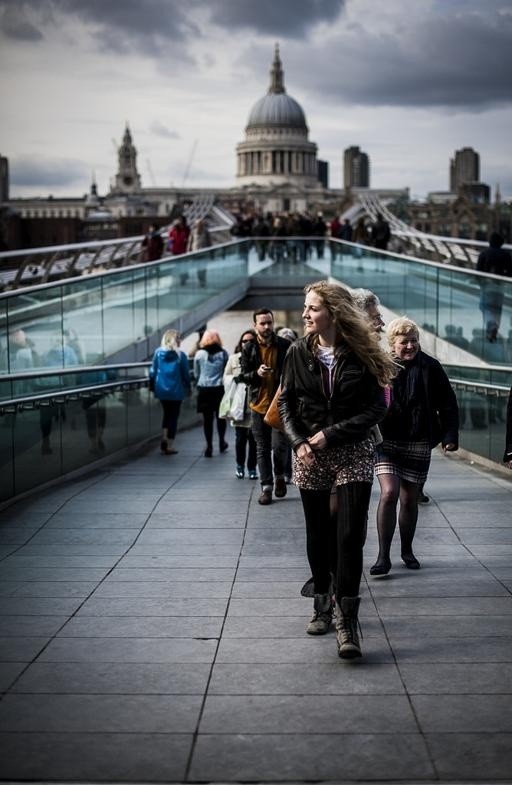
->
[334,594,363,658]
[301,573,334,635]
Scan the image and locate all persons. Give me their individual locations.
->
[141,207,394,290]
[0,326,121,455]
[370,315,459,575]
[351,286,384,337]
[278,280,408,657]
[149,310,299,505]
[474,230,511,342]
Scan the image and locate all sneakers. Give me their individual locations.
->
[161,429,429,505]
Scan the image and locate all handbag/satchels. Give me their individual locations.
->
[263,384,284,430]
[218,376,247,421]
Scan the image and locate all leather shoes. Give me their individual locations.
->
[370,559,391,575]
[401,553,420,568]
